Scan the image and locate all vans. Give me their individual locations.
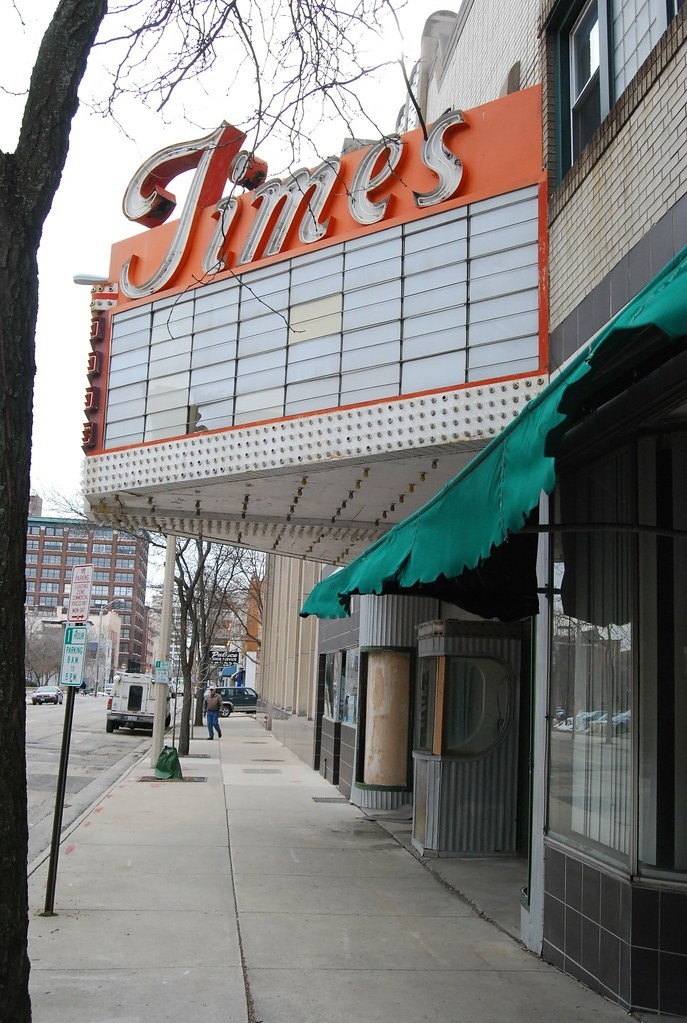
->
[104,670,177,734]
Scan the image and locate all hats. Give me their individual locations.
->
[207,686,215,689]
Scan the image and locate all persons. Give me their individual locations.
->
[204,687,223,740]
[82,682,87,696]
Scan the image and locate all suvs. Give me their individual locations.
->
[201,686,257,718]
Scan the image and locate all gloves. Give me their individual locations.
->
[218,711,223,717]
[203,711,206,718]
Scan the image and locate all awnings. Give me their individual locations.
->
[231,670,246,680]
[221,666,236,677]
[298,247,686,620]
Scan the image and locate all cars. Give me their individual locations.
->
[566,709,632,735]
[31,686,64,705]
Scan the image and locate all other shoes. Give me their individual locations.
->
[217,730,222,738]
[207,736,213,740]
